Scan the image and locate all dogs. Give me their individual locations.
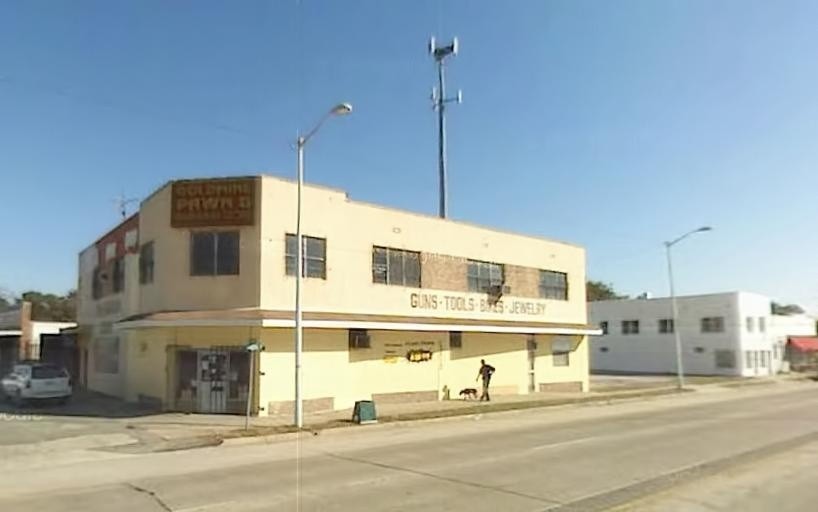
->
[460,388,477,401]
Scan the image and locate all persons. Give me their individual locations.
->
[475,358,495,402]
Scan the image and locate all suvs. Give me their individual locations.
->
[0,360,72,409]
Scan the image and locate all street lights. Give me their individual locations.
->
[292,100,354,423]
[660,224,712,390]
